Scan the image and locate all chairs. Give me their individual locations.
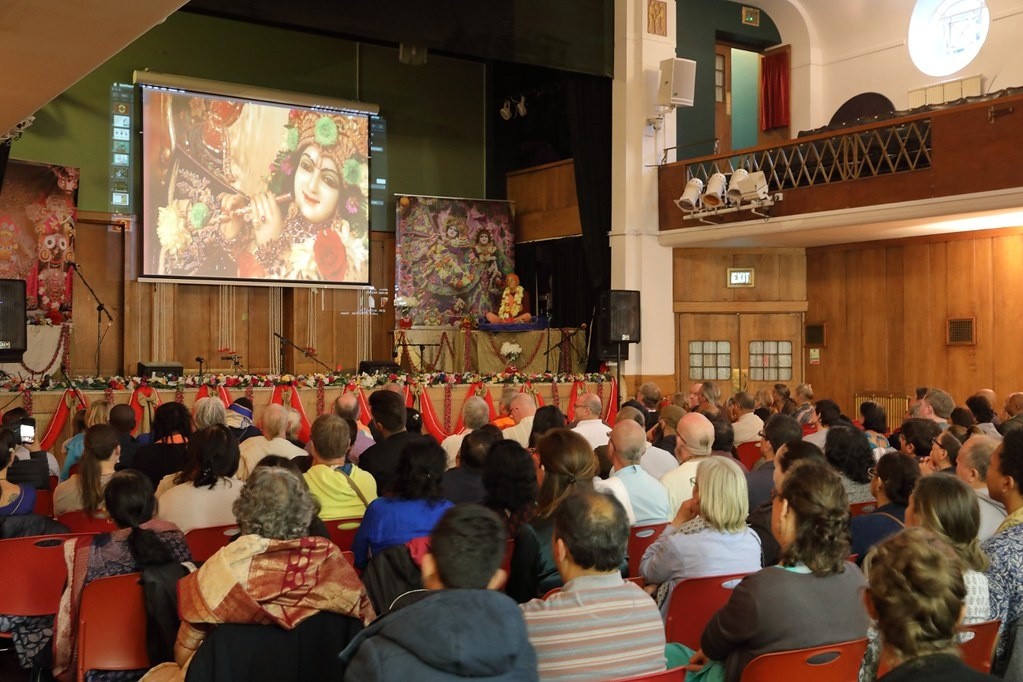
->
[0,419,1023,682]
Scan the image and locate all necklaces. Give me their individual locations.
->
[679,455,705,465]
[498,286,524,320]
[0,478,7,481]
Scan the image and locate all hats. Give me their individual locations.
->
[675,412,715,455]
[228,396,253,424]
[267,109,368,214]
[660,405,687,428]
[966,396,991,416]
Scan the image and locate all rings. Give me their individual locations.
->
[253,215,267,230]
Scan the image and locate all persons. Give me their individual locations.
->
[487,274,532,323]
[216,111,367,283]
[648,0,665,36]
[0,380,1023,682]
[405,209,511,326]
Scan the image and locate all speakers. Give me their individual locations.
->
[358,361,401,375]
[138,361,183,379]
[657,58,697,107]
[0,278,27,362]
[597,289,641,361]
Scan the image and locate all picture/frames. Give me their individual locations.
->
[648,0,668,38]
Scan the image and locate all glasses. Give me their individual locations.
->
[868,468,878,476]
[690,477,698,489]
[574,404,587,409]
[606,431,617,452]
[769,488,787,501]
[508,408,519,420]
[758,430,766,438]
[932,436,946,450]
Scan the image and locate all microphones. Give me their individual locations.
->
[65,261,80,266]
[195,357,204,361]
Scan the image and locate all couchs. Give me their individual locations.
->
[742,84,1023,189]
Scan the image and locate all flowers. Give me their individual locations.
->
[499,342,522,365]
[0,373,610,430]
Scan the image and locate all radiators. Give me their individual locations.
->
[854,391,911,433]
[908,73,981,108]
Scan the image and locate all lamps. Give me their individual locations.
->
[516,96,527,117]
[500,99,512,121]
[671,167,775,220]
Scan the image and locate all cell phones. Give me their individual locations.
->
[19,417,35,444]
